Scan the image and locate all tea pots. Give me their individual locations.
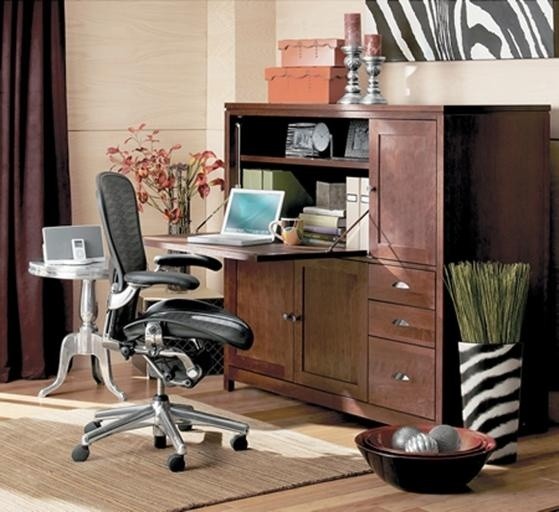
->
[268,218,305,246]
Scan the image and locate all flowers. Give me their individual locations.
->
[99,118,225,228]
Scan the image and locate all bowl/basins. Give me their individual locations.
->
[354,423,497,492]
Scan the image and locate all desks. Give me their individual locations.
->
[23,251,134,412]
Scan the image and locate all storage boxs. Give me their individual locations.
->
[258,63,351,106]
[274,39,350,71]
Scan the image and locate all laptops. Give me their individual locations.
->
[186,188,286,247]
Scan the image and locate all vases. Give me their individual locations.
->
[452,333,529,469]
[161,160,194,294]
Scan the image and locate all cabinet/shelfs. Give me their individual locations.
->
[217,93,554,442]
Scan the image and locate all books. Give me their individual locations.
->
[297,206,345,249]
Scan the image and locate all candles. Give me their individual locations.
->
[342,10,363,48]
[362,33,383,59]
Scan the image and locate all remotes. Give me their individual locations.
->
[72,239,86,259]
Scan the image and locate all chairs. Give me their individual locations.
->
[68,169,258,479]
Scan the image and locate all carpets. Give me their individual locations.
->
[1,367,376,511]
